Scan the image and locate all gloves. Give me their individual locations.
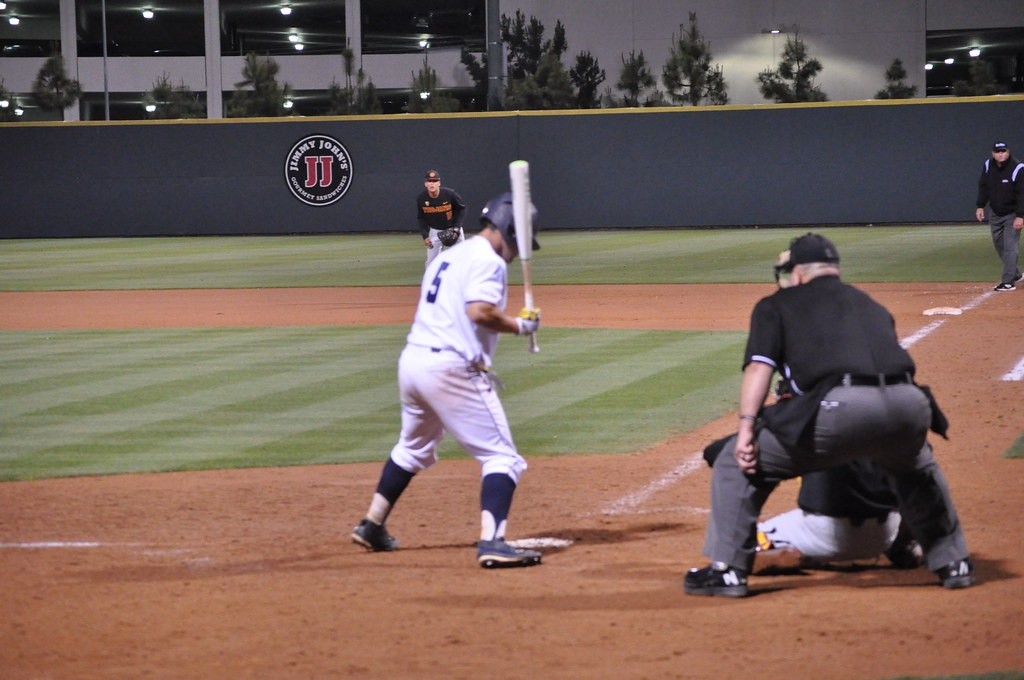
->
[518,307,541,337]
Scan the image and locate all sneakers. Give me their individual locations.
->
[478,542,543,568]
[1014,272,1024,284]
[351,519,402,551]
[993,282,1016,290]
[682,565,749,598]
[936,557,974,588]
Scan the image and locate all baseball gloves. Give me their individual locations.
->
[437,227,461,247]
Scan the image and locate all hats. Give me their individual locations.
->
[992,141,1008,152]
[424,170,441,182]
[790,232,839,267]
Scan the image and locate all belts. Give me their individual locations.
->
[838,371,904,387]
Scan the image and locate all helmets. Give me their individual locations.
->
[480,192,541,251]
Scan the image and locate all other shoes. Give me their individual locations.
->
[750,546,801,575]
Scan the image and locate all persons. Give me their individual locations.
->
[683,229,977,599]
[415,169,468,273]
[349,191,543,570]
[976,141,1024,292]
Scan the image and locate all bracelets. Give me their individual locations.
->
[738,414,758,426]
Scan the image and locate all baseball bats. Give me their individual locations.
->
[507,159,542,354]
